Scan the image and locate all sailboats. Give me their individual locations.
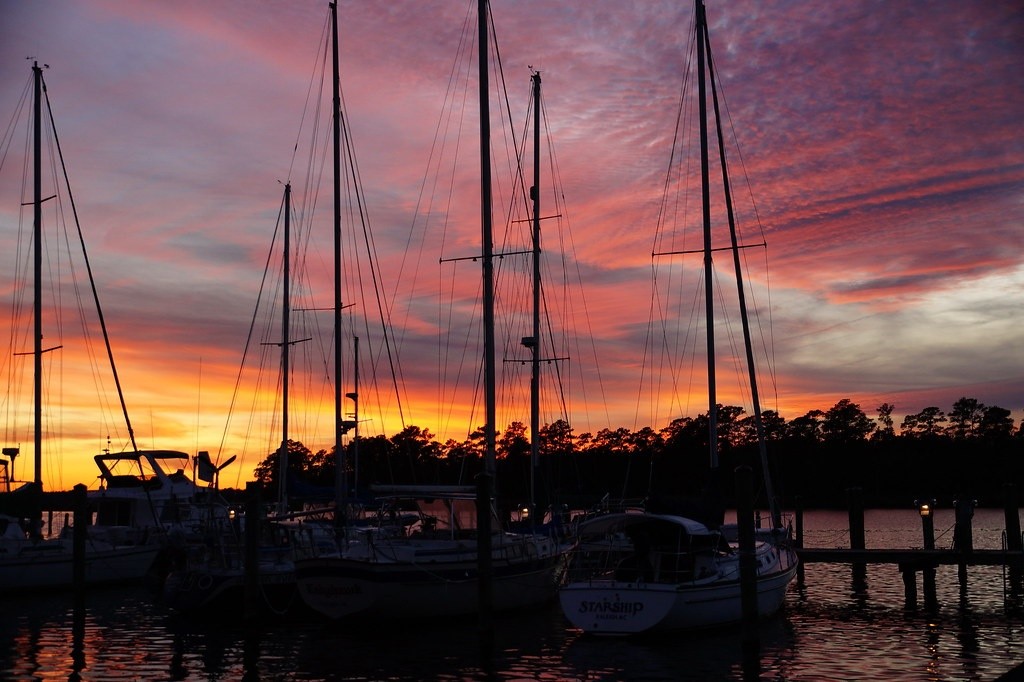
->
[0,0,581,633]
[551,0,799,642]
